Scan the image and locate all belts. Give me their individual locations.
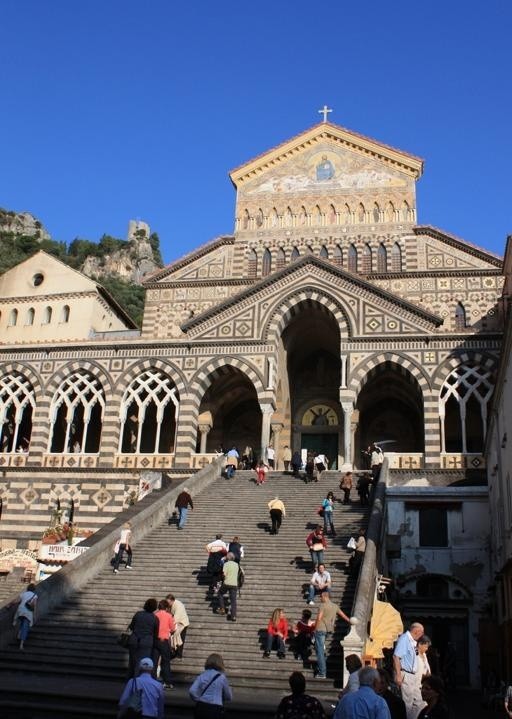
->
[400,668,414,674]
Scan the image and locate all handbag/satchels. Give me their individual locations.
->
[318,509,325,517]
[25,593,36,613]
[118,625,134,649]
[237,565,244,589]
[116,676,144,719]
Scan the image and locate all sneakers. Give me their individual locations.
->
[277,651,285,658]
[263,650,272,657]
[314,672,327,679]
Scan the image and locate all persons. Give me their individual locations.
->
[13,583,38,651]
[267,494,286,535]
[321,491,337,536]
[306,527,328,573]
[174,487,194,530]
[349,529,366,579]
[72,440,82,454]
[213,438,330,486]
[128,594,189,690]
[262,564,512,719]
[205,534,245,622]
[117,658,165,719]
[16,444,28,453]
[113,522,133,574]
[342,441,394,508]
[186,653,232,719]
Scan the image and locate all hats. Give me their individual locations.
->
[140,657,155,669]
